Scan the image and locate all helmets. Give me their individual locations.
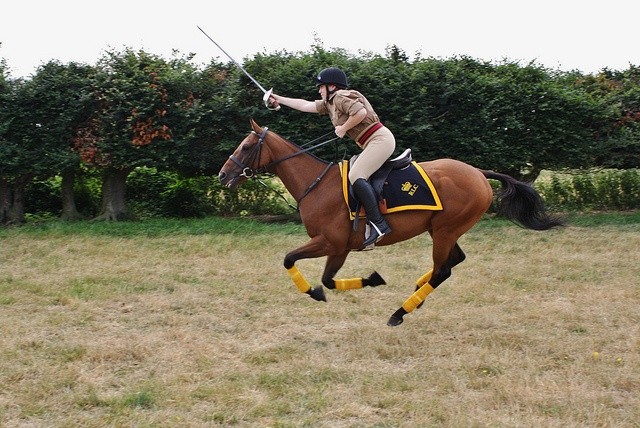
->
[311,66,350,103]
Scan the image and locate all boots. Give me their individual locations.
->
[351,178,393,247]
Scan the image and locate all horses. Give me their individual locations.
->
[218,117,569,326]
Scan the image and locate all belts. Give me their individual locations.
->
[355,122,385,149]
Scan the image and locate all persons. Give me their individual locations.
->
[269,67,396,247]
